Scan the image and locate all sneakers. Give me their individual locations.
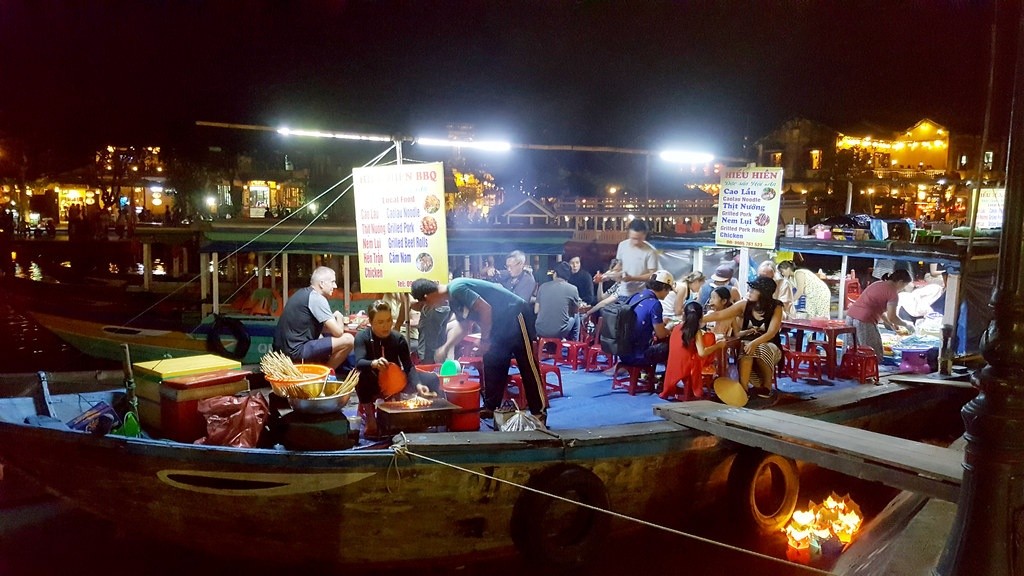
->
[744,390,750,398]
[757,387,773,398]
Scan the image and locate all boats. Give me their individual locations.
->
[0,221,1001,576]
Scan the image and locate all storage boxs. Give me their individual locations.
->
[131,353,243,434]
[159,370,252,444]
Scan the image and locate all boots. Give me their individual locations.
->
[366,404,378,436]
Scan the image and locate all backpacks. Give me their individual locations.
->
[600,293,658,355]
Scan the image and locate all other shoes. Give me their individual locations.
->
[644,375,659,383]
[480,410,494,418]
[657,380,680,393]
[531,413,546,428]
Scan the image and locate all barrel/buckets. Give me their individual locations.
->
[442,379,482,432]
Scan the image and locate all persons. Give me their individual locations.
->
[0,197,184,243]
[255,200,293,219]
[354,300,440,437]
[413,277,549,427]
[482,220,832,403]
[841,269,915,380]
[272,266,354,371]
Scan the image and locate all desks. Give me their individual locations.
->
[780,319,857,380]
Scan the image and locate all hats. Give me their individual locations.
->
[649,270,678,294]
[746,277,777,295]
[711,265,734,282]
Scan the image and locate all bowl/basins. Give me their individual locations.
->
[264,365,329,398]
[281,381,356,411]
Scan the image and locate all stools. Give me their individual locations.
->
[459,334,878,412]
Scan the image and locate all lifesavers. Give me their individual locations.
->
[732,452,799,534]
[209,317,250,359]
[512,464,613,561]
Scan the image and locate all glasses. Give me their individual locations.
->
[504,261,520,269]
